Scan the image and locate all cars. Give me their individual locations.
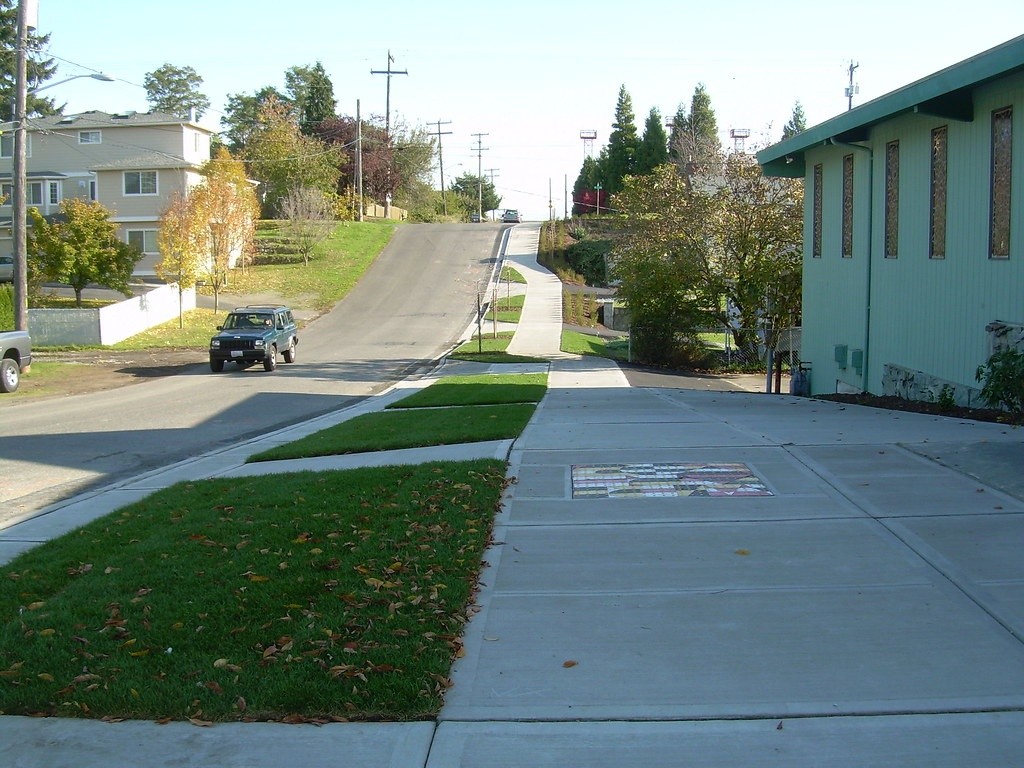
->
[502,210,523,223]
[472,214,479,223]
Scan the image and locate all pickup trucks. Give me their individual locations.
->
[1,330,32,393]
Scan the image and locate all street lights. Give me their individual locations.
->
[440,164,464,216]
[479,175,500,221]
[13,75,114,330]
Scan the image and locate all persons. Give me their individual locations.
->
[264,318,274,327]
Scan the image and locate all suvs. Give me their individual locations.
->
[209,304,299,372]
